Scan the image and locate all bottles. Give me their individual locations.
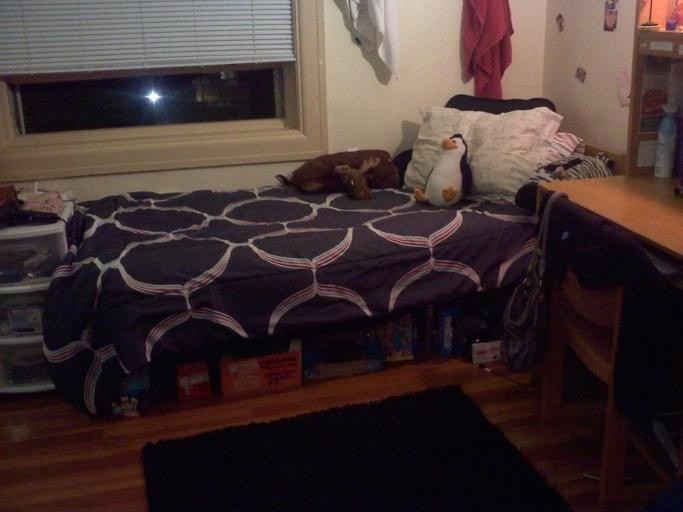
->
[654,105,678,179]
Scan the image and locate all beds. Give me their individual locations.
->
[67,143,625,421]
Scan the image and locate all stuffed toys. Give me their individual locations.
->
[298,149,402,200]
[414,132,474,209]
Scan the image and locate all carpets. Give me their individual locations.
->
[140,384,572,512]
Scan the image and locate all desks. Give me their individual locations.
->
[532,176,683,511]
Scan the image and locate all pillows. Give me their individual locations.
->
[401,94,586,206]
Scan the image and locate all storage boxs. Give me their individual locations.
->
[1,204,68,395]
[218,349,303,394]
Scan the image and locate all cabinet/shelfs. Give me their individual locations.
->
[626,1,682,179]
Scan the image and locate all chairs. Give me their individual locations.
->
[541,189,672,509]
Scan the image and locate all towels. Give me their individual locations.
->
[346,0,516,100]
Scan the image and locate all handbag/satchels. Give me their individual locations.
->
[500,190,568,373]
